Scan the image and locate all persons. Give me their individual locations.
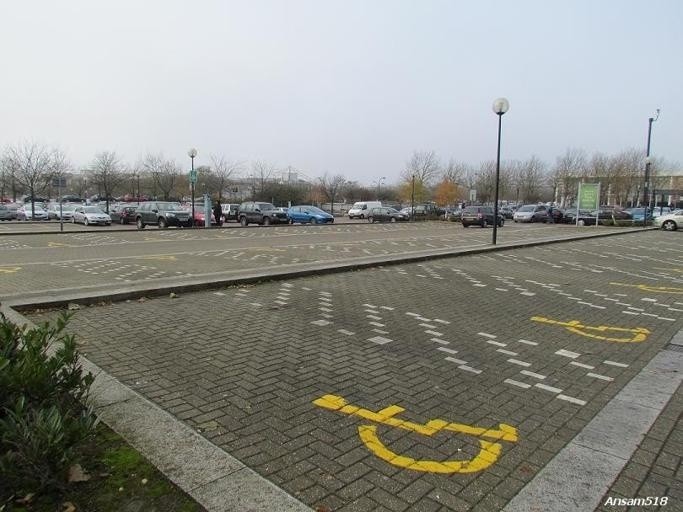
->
[211,200,222,224]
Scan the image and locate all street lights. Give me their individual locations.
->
[201,182,205,199]
[644,155,655,226]
[411,171,417,220]
[491,97,512,244]
[378,177,385,191]
[187,146,198,227]
[82,175,88,205]
[135,171,141,205]
